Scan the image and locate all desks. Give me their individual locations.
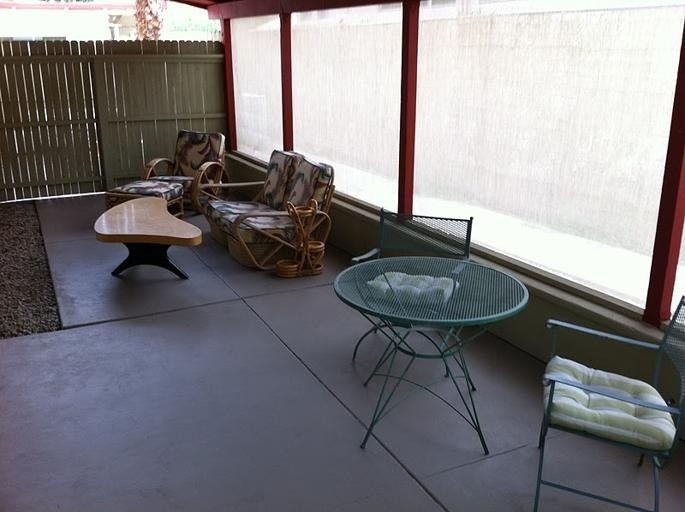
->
[332,256,530,456]
[94,196,203,280]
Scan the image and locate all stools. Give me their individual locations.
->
[106,180,185,220]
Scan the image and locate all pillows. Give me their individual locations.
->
[260,149,305,209]
[281,158,331,211]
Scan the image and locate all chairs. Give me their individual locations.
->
[142,129,233,214]
[532,295,684,511]
[351,207,473,378]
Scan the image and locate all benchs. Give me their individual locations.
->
[199,149,335,278]
